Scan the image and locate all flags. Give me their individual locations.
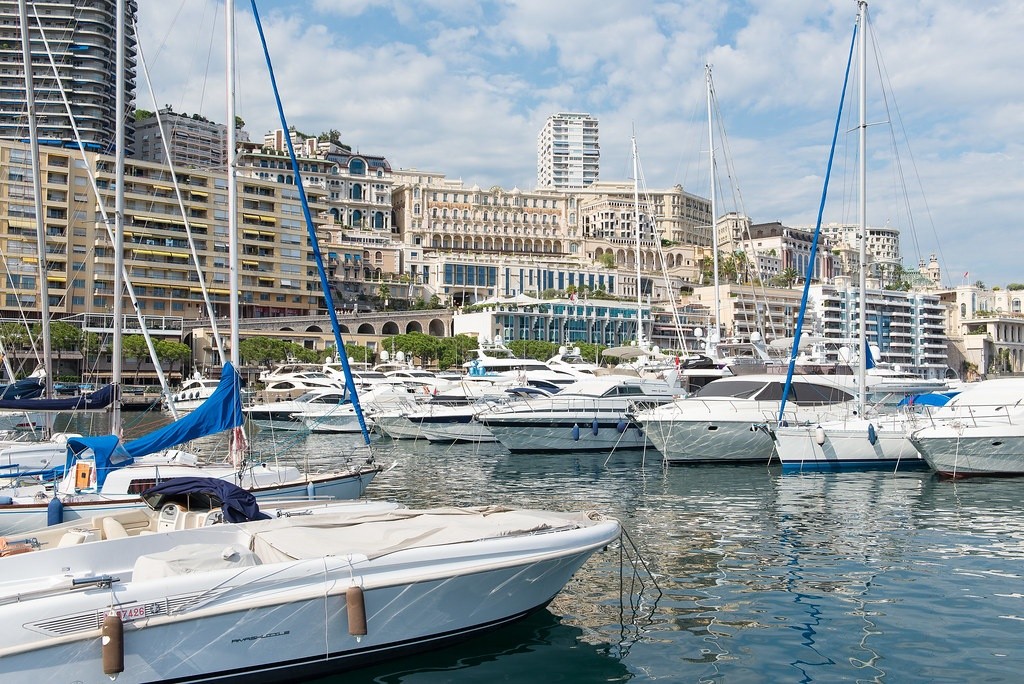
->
[963,271,969,278]
[569,291,578,306]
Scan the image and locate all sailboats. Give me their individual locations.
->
[0,0,624,684]
[603,0,1024,480]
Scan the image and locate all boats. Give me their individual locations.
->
[165,334,687,449]
